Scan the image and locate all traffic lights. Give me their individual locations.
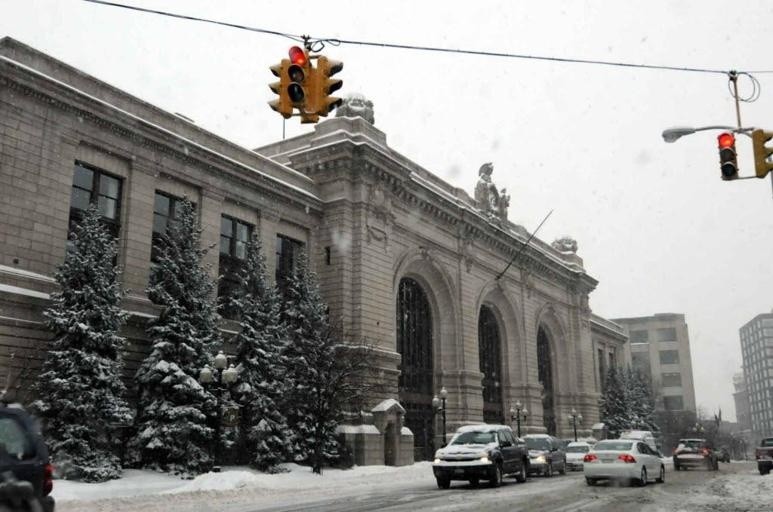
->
[267,46,343,123]
[717,132,739,180]
[752,129,773,177]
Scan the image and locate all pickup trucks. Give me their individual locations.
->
[755,437,773,473]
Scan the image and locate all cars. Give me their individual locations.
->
[673,438,730,470]
[0,398,55,511]
[433,424,529,488]
[516,432,601,477]
[584,439,665,486]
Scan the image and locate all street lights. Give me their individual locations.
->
[432,386,447,461]
[201,351,238,473]
[568,408,583,440]
[511,400,527,437]
